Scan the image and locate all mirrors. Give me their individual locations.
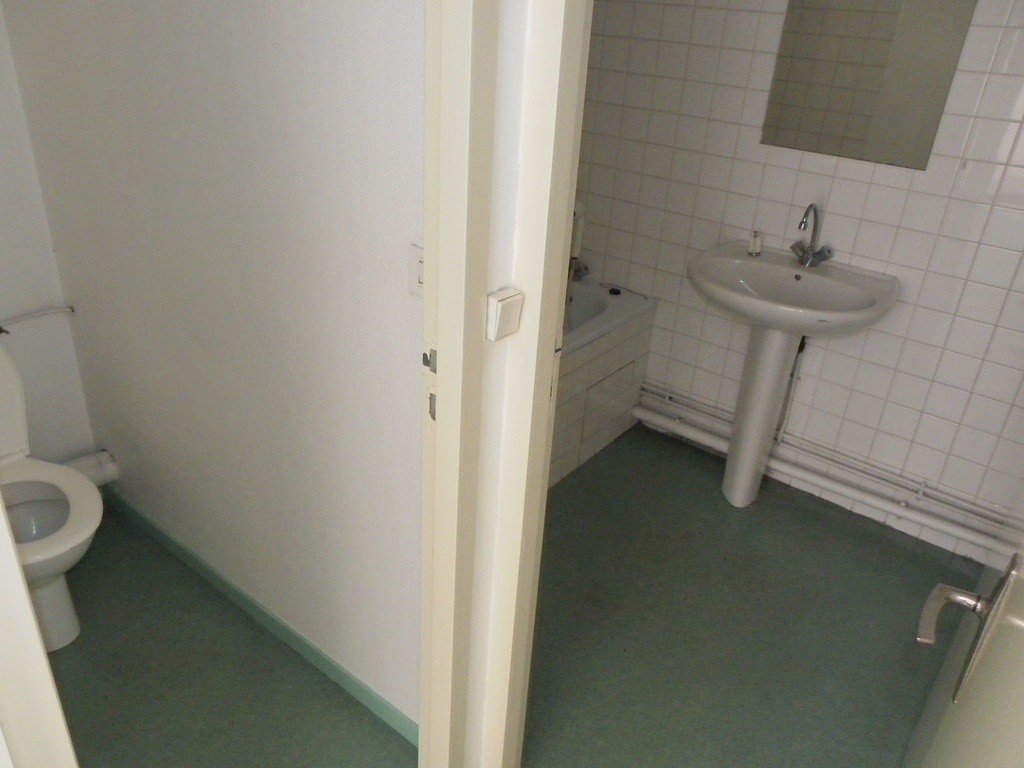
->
[759,0,978,171]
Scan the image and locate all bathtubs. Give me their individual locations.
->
[559,272,660,359]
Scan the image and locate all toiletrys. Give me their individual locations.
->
[746,229,762,257]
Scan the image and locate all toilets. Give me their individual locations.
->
[0,344,104,654]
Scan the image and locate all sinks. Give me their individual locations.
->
[685,238,901,339]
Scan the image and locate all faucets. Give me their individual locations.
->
[789,202,834,268]
